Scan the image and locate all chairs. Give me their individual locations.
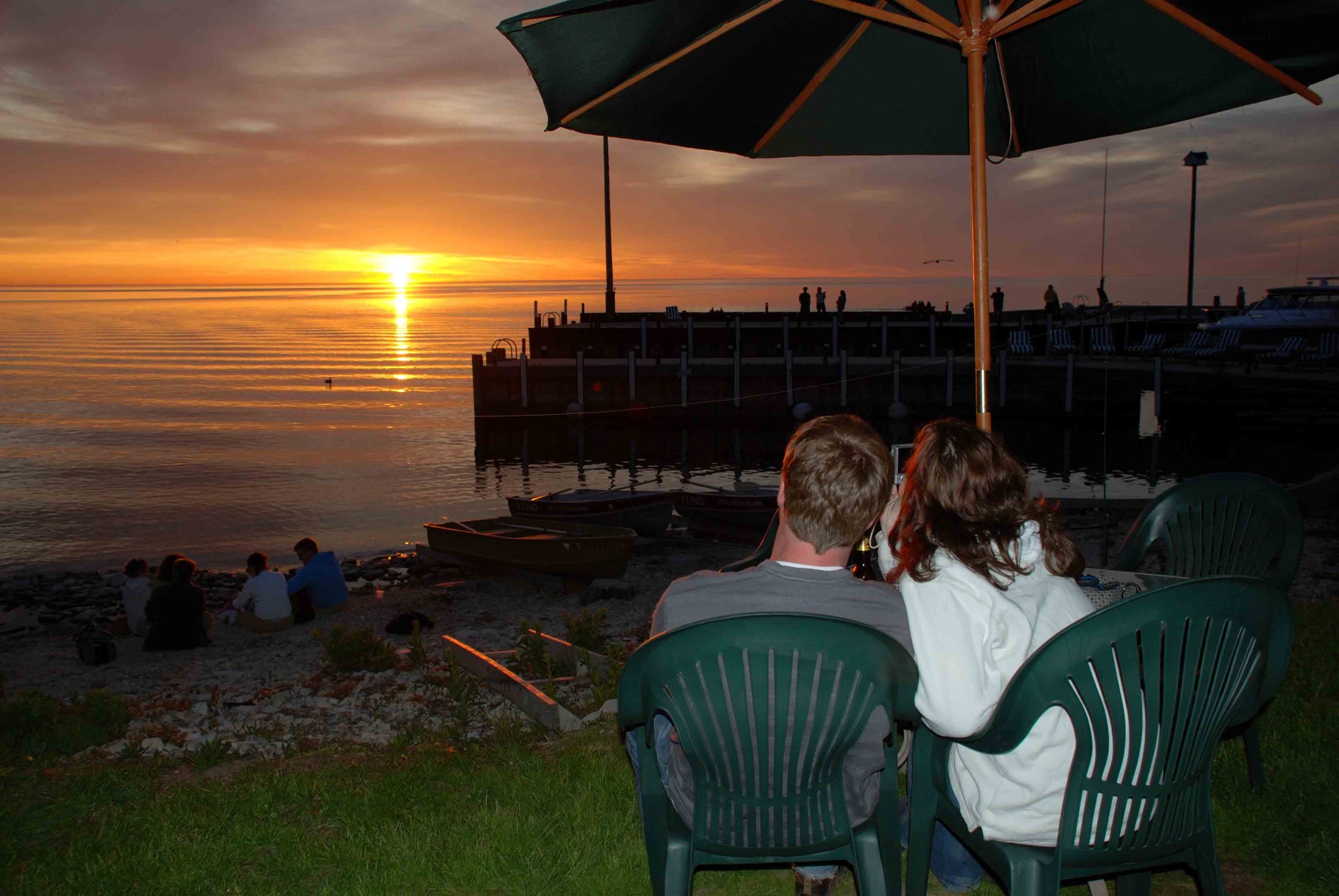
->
[1106,472,1303,786]
[617,610,923,896]
[905,574,1296,896]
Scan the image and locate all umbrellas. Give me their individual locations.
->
[495,0,1339,451]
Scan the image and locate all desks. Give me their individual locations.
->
[1076,566,1147,612]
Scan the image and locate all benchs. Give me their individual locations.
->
[516,532,569,540]
[478,528,523,535]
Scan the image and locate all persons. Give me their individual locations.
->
[876,418,1166,891]
[121,553,213,651]
[1236,286,1246,308]
[962,302,974,314]
[799,287,811,315]
[990,287,1004,312]
[231,553,295,630]
[286,537,350,614]
[1044,285,1060,309]
[814,286,827,313]
[835,290,846,312]
[625,414,918,896]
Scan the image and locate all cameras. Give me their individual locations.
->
[891,443,917,484]
